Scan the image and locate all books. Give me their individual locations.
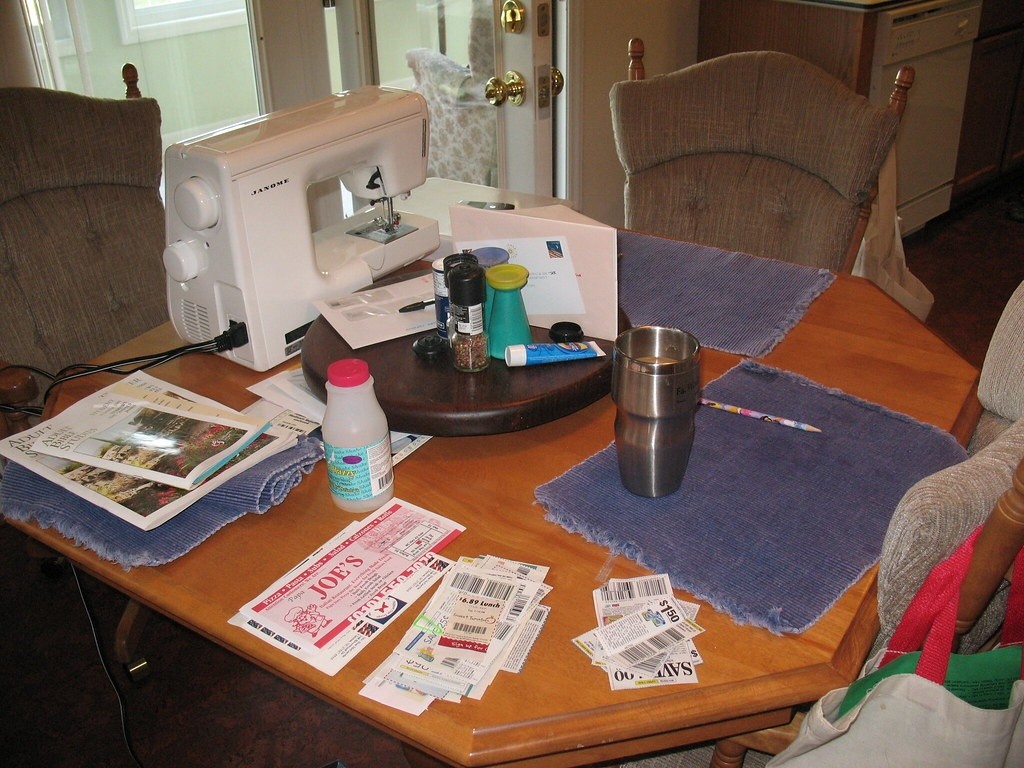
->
[0,368,298,531]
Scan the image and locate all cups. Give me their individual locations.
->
[432,257,451,339]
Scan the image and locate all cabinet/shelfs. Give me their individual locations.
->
[950,0,1024,197]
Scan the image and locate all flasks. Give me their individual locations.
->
[611,325,700,498]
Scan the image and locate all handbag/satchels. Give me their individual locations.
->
[760,524,1024,766]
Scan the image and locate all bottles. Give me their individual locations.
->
[471,245,511,359]
[447,263,492,373]
[485,263,533,361]
[321,359,394,514]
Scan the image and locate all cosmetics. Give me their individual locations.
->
[505,339,607,367]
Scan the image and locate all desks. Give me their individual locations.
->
[0,226,982,768]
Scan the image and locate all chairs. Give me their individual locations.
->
[0,63,164,577]
[609,454,1024,768]
[608,37,917,275]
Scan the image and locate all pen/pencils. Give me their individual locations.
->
[399,298,435,313]
[698,398,822,432]
